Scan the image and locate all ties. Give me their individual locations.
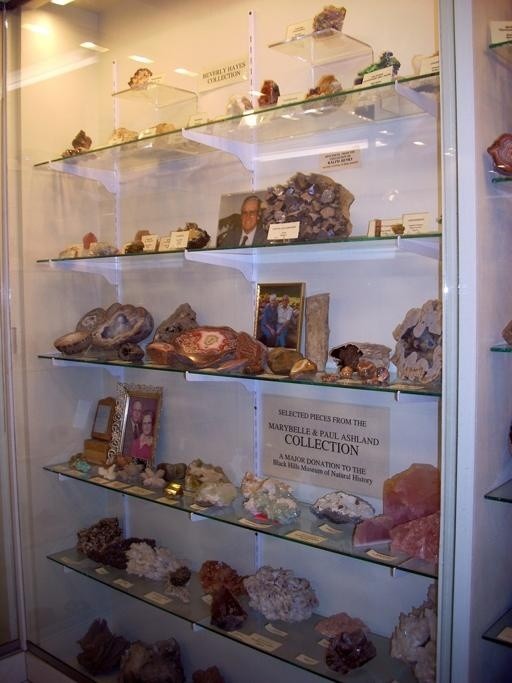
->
[241,235,248,246]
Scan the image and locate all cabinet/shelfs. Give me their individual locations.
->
[0,1,512,683]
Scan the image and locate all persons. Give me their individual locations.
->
[259,293,278,347]
[130,410,154,461]
[217,194,270,247]
[122,399,143,458]
[274,294,294,346]
